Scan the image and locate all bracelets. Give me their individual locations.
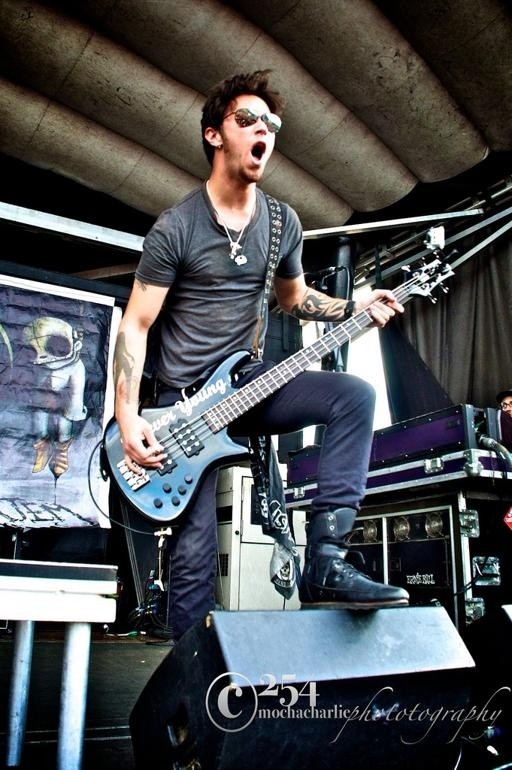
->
[344,299,356,320]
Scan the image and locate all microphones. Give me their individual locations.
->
[303,265,345,286]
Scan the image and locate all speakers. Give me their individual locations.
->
[459,603,512,654]
[127,606,479,769]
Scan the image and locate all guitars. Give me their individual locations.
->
[98,255,455,532]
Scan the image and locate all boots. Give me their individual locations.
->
[299,507,410,610]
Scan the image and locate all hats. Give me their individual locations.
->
[496,389,512,403]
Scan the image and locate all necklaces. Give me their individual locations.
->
[206,180,255,268]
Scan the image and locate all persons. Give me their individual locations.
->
[496,389,512,417]
[111,68,410,645]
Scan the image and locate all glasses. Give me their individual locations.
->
[223,108,282,133]
